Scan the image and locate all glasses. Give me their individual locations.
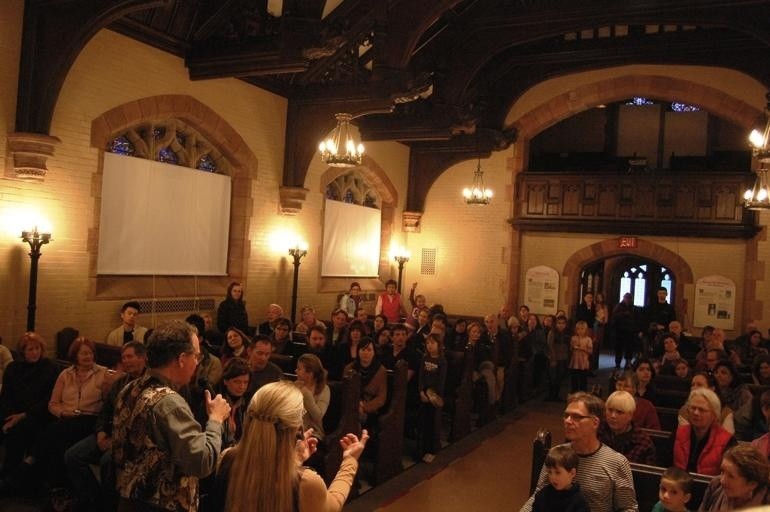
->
[560,412,595,421]
[188,352,205,364]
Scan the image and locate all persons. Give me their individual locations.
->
[0,281,770,512]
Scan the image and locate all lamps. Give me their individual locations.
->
[742,163,770,212]
[288,248,308,329]
[749,116,770,163]
[462,156,492,206]
[394,255,410,295]
[318,113,364,169]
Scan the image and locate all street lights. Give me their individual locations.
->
[285,236,312,327]
[392,246,411,293]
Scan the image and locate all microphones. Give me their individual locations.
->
[198,377,216,399]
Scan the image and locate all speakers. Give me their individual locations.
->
[58,327,79,360]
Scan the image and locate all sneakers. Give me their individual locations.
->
[422,453,437,463]
